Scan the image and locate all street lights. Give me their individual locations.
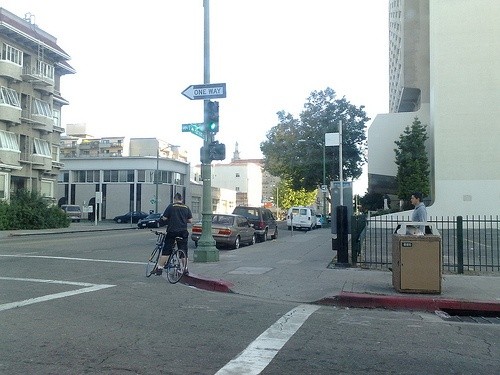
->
[273,184,278,218]
[298,139,328,227]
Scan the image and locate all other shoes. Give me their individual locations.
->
[149,267,162,275]
[184,271,189,275]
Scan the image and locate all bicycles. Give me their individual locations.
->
[145,228,188,284]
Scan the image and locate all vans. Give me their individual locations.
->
[286,208,317,231]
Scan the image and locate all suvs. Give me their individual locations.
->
[231,204,278,242]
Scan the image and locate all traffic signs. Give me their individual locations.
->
[181,123,204,132]
[180,83,227,99]
[190,124,208,141]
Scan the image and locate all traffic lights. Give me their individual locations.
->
[204,101,220,132]
[209,143,227,161]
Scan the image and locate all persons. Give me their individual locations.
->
[150,192,193,279]
[388,193,427,271]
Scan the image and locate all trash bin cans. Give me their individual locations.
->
[391,222,443,295]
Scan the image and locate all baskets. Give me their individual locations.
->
[155,235,167,245]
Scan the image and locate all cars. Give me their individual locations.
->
[136,212,167,230]
[62,205,82,223]
[190,214,257,250]
[113,211,150,223]
[315,214,323,228]
[327,213,332,224]
